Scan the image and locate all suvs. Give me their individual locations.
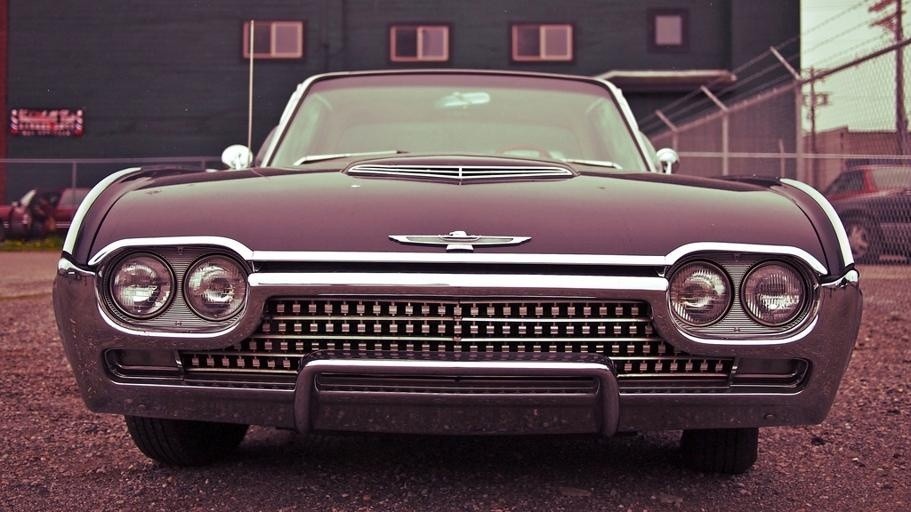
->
[0,184,90,238]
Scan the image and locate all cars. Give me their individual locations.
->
[823,165,910,201]
[819,188,911,265]
[49,69,872,480]
